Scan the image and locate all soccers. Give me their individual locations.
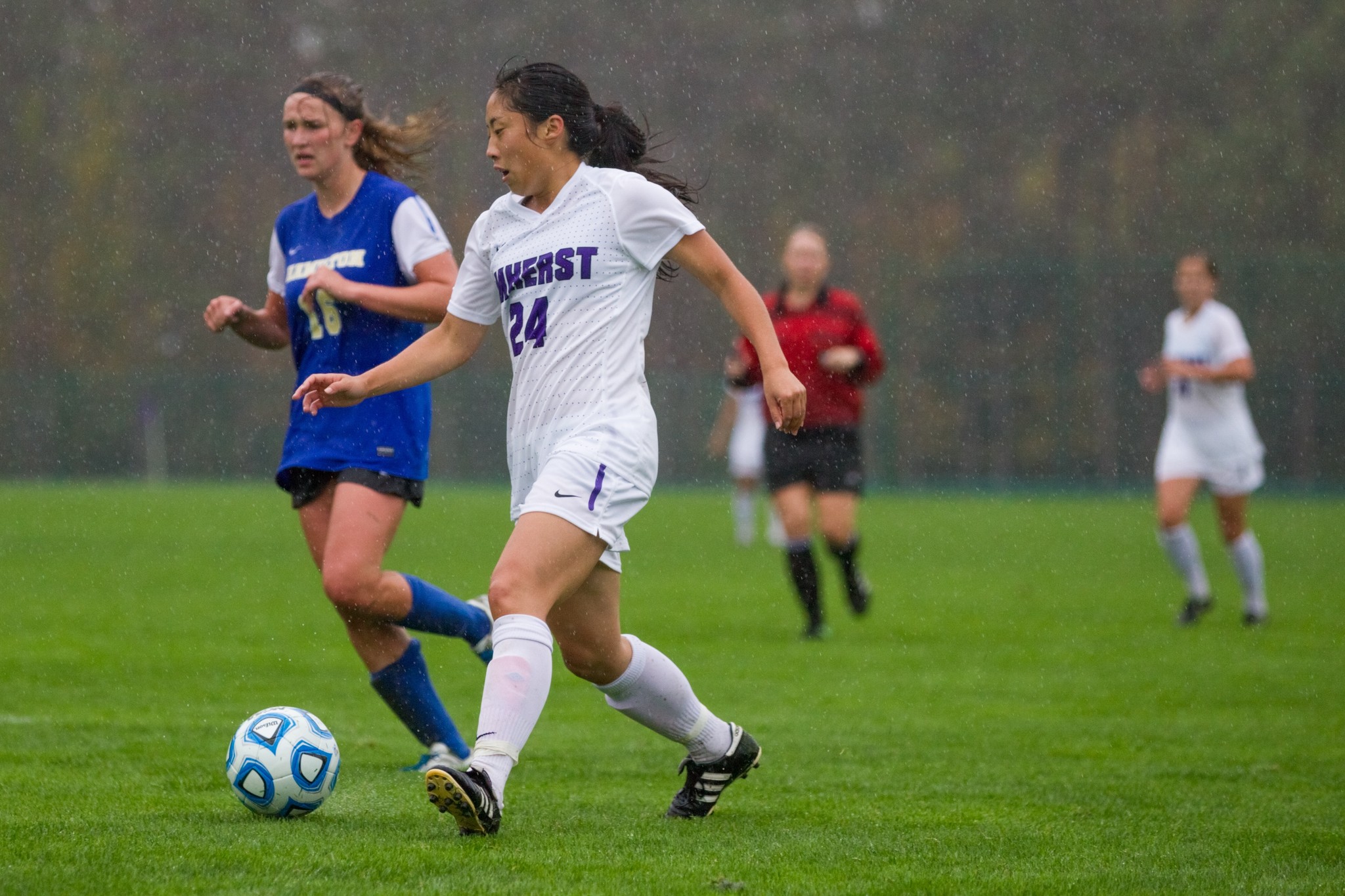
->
[224,705,341,818]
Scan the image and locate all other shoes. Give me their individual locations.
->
[806,621,821,638]
[847,577,868,614]
[1241,613,1259,627]
[1181,593,1214,623]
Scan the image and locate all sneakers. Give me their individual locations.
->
[466,593,493,663]
[667,722,761,819]
[426,765,500,836]
[405,742,475,773]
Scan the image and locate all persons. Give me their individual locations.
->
[205,70,495,774]
[1136,248,1270,627]
[724,223,885,639]
[712,357,786,547]
[289,65,806,836]
